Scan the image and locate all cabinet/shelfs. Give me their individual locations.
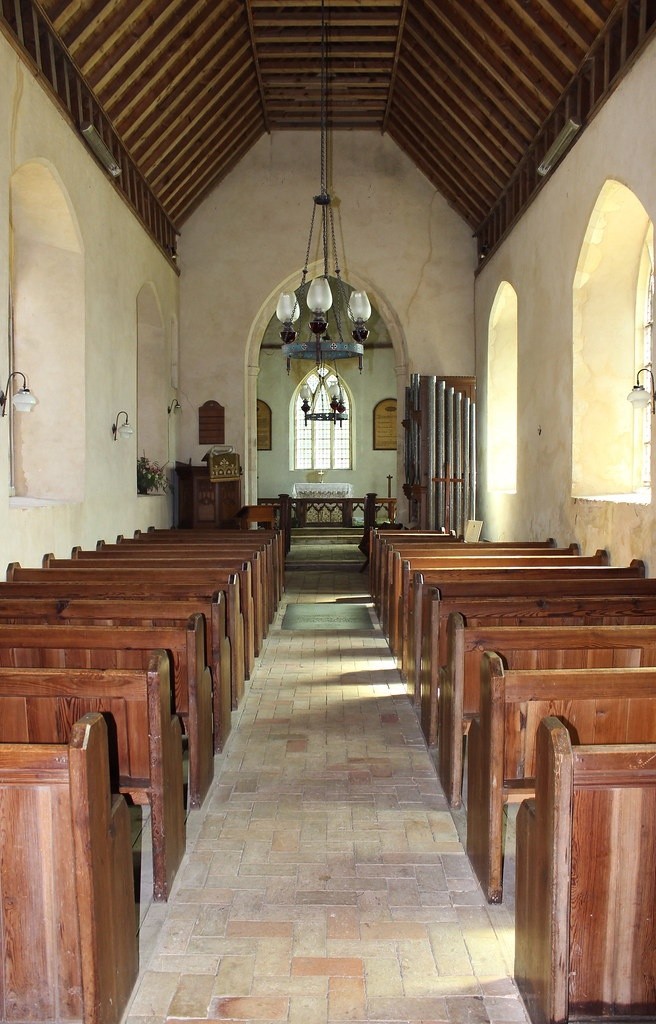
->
[174,466,241,529]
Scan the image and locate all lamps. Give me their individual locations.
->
[628,369,656,412]
[168,399,180,414]
[113,411,134,441]
[301,312,348,426]
[0,372,36,417]
[536,115,582,177]
[273,0,374,375]
[80,120,123,177]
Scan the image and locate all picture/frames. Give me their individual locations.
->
[257,399,272,451]
[372,399,398,450]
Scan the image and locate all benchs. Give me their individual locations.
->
[366,522,656,1024]
[0,526,284,1024]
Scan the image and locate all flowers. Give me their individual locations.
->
[137,457,174,493]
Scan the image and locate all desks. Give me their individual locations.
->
[291,482,352,522]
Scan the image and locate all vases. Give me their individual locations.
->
[137,485,147,494]
